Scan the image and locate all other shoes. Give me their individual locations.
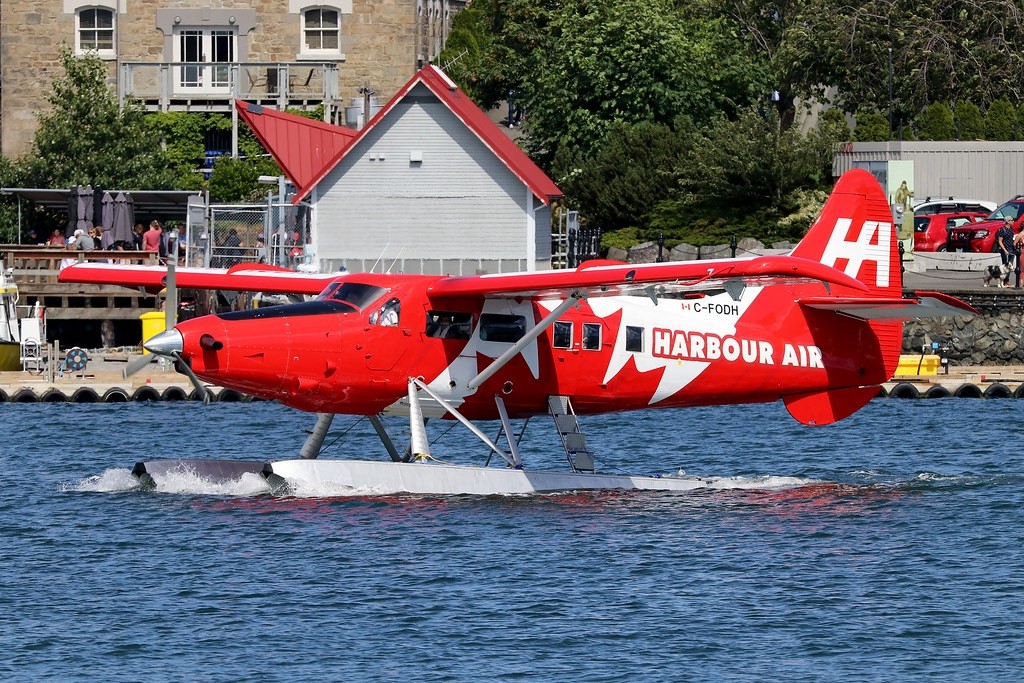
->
[1004,282,1015,287]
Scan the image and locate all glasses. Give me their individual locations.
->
[88,231,94,234]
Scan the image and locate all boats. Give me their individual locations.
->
[0,258,48,371]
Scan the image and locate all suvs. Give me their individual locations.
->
[911,206,986,253]
[910,197,1004,228]
[946,192,1024,256]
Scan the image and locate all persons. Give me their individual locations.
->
[998,216,1024,288]
[49,220,305,270]
[428,314,461,340]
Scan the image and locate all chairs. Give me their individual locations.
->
[486,324,523,341]
[427,323,469,338]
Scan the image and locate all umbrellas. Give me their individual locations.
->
[66,184,134,251]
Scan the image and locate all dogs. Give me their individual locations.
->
[983,262,1014,288]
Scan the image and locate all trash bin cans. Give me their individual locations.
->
[139,311,180,355]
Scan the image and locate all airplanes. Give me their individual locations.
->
[53,168,979,502]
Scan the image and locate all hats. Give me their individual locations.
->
[68,236,76,244]
[29,230,35,236]
[1004,216,1013,223]
[78,228,84,233]
[74,230,81,238]
